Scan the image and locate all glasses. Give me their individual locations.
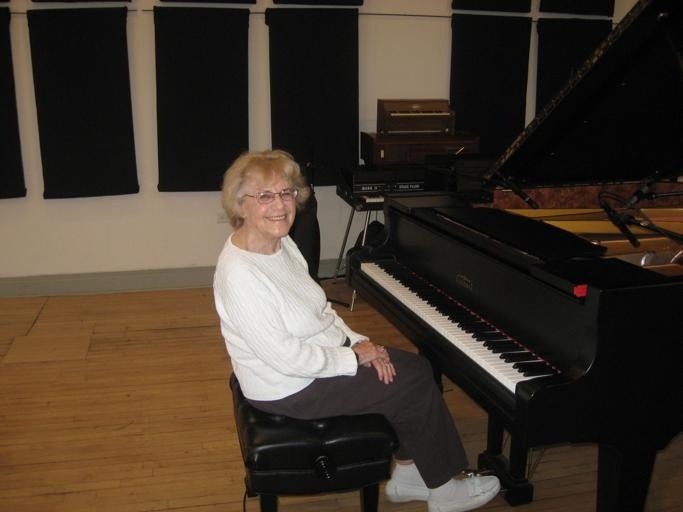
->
[247,188,297,204]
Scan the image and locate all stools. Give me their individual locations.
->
[228,371,395,512]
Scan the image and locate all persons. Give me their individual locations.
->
[211,146,501,511]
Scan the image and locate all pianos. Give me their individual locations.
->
[335,0,681,510]
[336,160,499,310]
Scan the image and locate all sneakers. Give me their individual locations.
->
[428,476,501,512]
[386,477,429,503]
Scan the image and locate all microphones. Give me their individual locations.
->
[622,181,655,210]
[599,202,640,248]
[508,183,539,209]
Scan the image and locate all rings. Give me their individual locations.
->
[381,345,384,351]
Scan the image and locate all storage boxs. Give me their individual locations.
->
[360,130,481,167]
